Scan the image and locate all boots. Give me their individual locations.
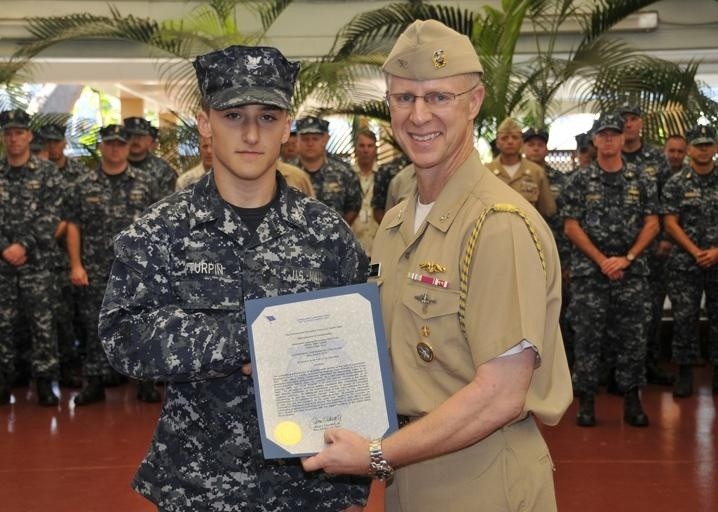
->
[137,381,162,403]
[672,365,694,397]
[623,390,649,427]
[576,394,595,427]
[646,362,676,386]
[59,374,83,389]
[9,361,32,385]
[108,374,129,386]
[74,376,105,405]
[36,381,59,406]
[0,383,11,404]
[712,365,718,395]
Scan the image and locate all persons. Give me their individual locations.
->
[95,44,371,512]
[0,107,212,407]
[483,107,718,427]
[298,17,576,511]
[277,115,411,260]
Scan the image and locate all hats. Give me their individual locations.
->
[151,127,159,137]
[381,18,485,81]
[0,108,31,131]
[496,117,523,135]
[523,127,549,144]
[39,123,66,141]
[321,119,329,133]
[594,111,624,135]
[575,133,587,152]
[29,128,48,153]
[193,43,301,111]
[296,116,324,136]
[123,117,151,137]
[100,124,131,145]
[619,103,642,117]
[686,124,716,145]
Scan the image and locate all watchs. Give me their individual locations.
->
[367,439,394,483]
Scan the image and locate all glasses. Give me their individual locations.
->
[383,87,477,108]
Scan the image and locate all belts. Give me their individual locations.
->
[393,415,427,430]
